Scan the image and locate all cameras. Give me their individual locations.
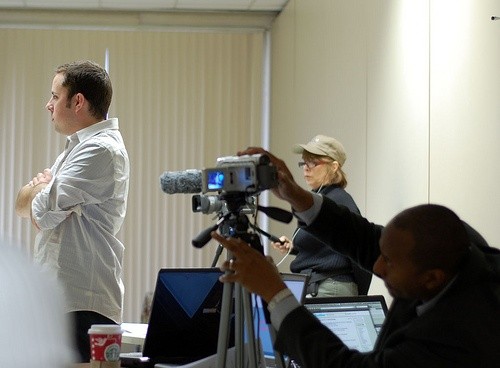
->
[200,155,278,194]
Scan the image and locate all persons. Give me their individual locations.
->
[211,147,500,368]
[15,61,130,364]
[215,171,222,186]
[270,134,373,298]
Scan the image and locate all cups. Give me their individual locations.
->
[88,324,124,368]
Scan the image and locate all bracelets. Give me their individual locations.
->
[268,288,293,312]
[33,181,49,188]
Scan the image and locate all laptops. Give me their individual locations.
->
[120,268,237,368]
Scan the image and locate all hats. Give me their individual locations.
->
[294,135,346,167]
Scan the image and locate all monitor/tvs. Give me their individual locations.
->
[302,294,389,354]
[245,272,309,367]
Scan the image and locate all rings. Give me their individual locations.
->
[229,259,234,270]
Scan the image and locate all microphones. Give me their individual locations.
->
[160,170,202,195]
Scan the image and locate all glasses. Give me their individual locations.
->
[299,162,330,169]
